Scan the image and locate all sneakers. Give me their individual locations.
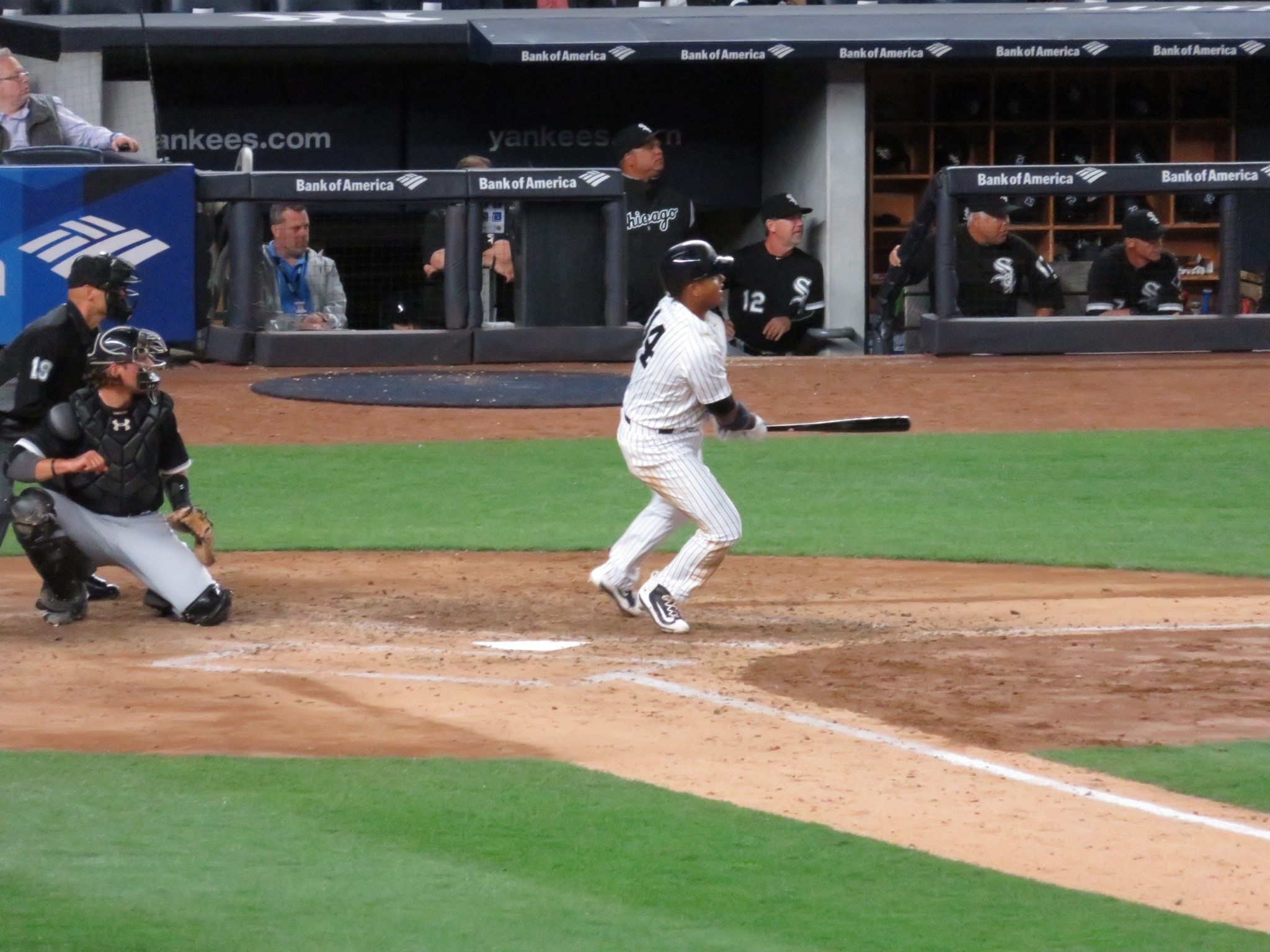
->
[639,581,689,633]
[591,568,640,615]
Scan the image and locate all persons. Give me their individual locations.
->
[588,240,767,634]
[889,196,1066,316]
[0,48,139,152]
[0,252,142,600]
[1085,210,1184,316]
[379,291,424,330]
[422,156,514,322]
[727,192,825,356]
[262,201,347,331]
[594,123,735,337]
[3,326,233,627]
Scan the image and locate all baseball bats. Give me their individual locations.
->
[766,416,912,432]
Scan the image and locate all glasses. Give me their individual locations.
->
[0,69,30,82]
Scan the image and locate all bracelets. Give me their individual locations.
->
[51,459,58,478]
[491,254,497,269]
[110,132,128,142]
[1130,307,1136,315]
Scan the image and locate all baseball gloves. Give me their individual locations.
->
[167,506,216,566]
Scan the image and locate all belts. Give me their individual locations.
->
[624,415,673,434]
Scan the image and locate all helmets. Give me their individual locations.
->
[662,240,734,291]
[82,325,152,380]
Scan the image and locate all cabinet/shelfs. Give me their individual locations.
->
[867,67,1238,296]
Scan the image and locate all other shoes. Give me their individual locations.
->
[102,585,120,599]
[42,604,86,625]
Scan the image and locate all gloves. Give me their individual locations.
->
[743,413,768,442]
[710,415,732,441]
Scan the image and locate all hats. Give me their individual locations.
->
[762,192,813,222]
[67,256,129,287]
[1123,209,1167,241]
[968,195,1020,216]
[612,123,667,164]
